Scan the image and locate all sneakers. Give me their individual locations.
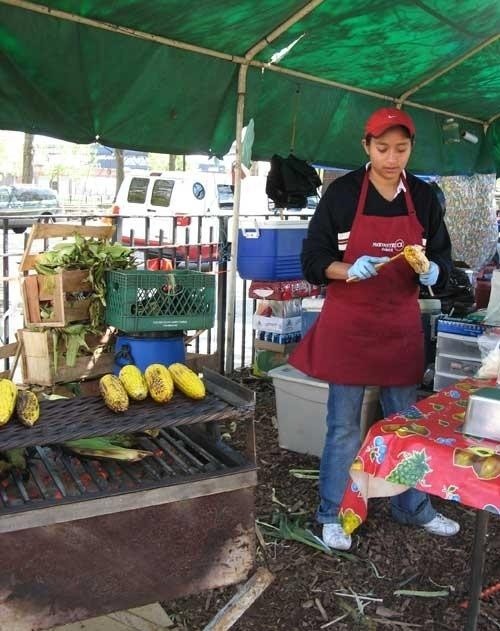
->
[416,512,461,538]
[322,522,352,551]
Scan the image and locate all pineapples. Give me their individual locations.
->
[385,448,434,488]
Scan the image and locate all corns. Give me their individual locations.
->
[15,390,40,427]
[144,364,174,404]
[404,245,430,274]
[99,374,129,413]
[119,364,148,401]
[168,363,206,399]
[0,378,18,426]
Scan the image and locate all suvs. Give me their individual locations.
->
[0,184,59,234]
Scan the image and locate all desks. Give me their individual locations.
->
[370,376,500,629]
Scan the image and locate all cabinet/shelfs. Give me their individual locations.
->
[432,332,481,392]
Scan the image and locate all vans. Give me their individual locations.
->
[112,172,322,263]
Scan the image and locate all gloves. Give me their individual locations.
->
[420,260,440,286]
[347,255,390,282]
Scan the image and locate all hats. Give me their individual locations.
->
[363,108,415,140]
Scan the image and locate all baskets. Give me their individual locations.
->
[105,268,217,334]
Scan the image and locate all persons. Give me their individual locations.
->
[286,107,461,552]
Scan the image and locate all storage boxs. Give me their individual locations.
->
[238,221,309,282]
[23,223,113,402]
[268,296,441,459]
